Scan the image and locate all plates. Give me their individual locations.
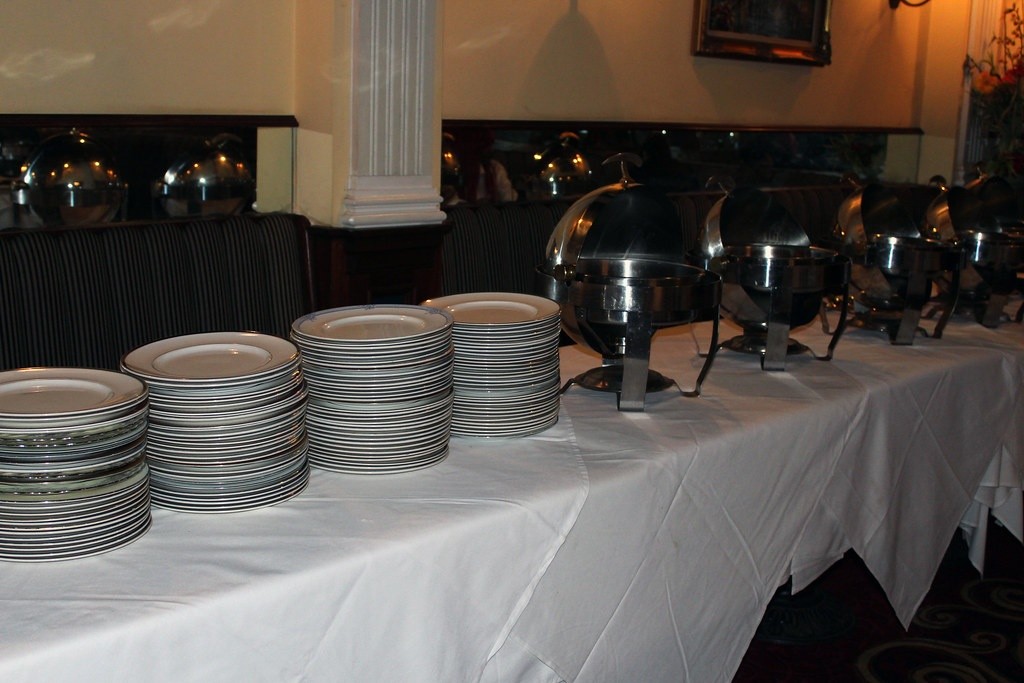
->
[0,292,562,564]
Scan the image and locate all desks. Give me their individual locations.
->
[0,299,1024,683]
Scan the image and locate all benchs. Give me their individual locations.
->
[441,163,927,345]
[0,214,318,373]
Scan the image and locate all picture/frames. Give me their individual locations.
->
[692,0,833,65]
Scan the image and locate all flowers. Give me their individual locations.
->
[964,2,1024,186]
